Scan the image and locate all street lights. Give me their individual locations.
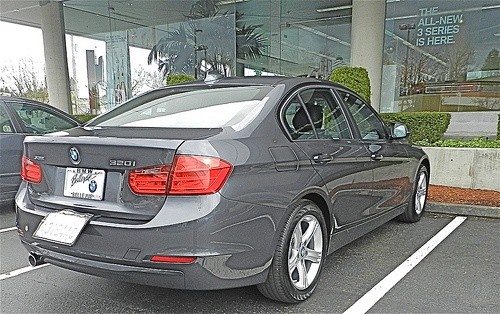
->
[398,21,416,114]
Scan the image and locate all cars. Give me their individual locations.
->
[0,95,85,206]
[14,73,432,304]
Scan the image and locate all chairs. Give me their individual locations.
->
[293,103,333,140]
[1,120,16,132]
